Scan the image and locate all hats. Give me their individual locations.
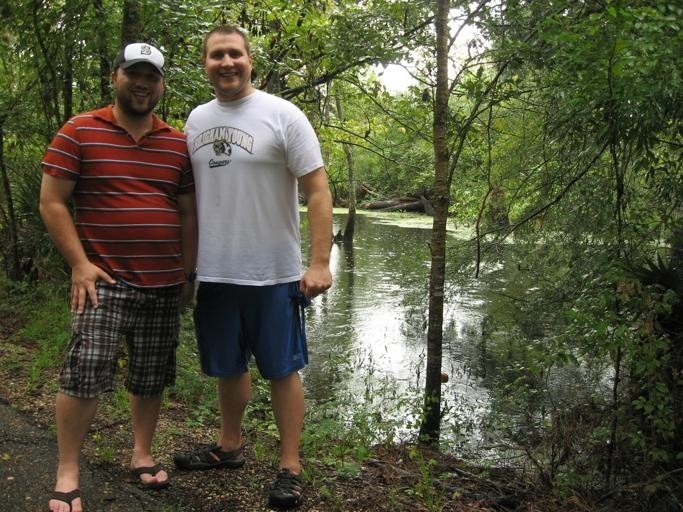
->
[113,42,165,77]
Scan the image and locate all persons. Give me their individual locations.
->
[37,41,198,512]
[184,24,334,507]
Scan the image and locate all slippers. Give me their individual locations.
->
[48,489,83,512]
[129,462,169,488]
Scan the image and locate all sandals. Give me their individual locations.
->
[268,463,309,510]
[172,445,248,472]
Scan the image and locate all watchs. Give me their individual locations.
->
[184,268,198,283]
